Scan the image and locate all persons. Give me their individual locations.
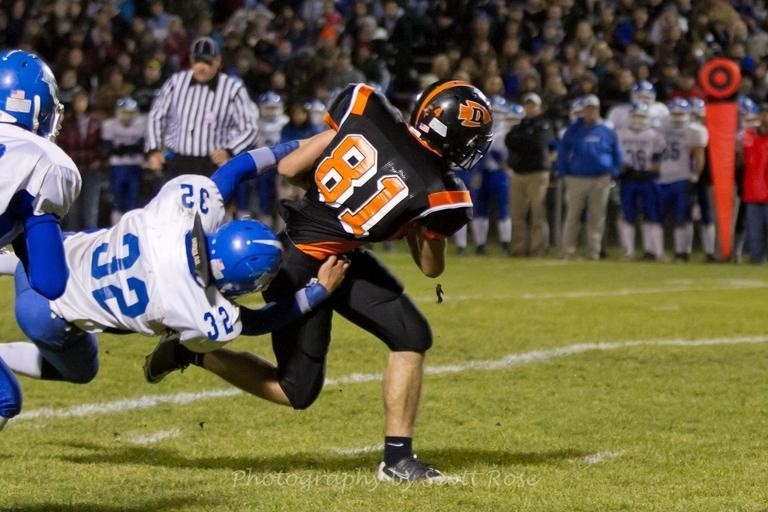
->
[2,1,768,268]
[0,46,84,432]
[1,133,352,386]
[141,79,495,486]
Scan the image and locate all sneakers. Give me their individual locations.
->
[143,331,193,382]
[376,457,461,486]
[455,244,766,264]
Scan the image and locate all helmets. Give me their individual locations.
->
[408,79,495,172]
[207,218,286,298]
[491,94,524,120]
[1,47,64,138]
[115,97,140,121]
[260,92,287,119]
[630,79,759,122]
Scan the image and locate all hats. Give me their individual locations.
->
[582,95,600,109]
[190,36,220,64]
[523,93,541,107]
